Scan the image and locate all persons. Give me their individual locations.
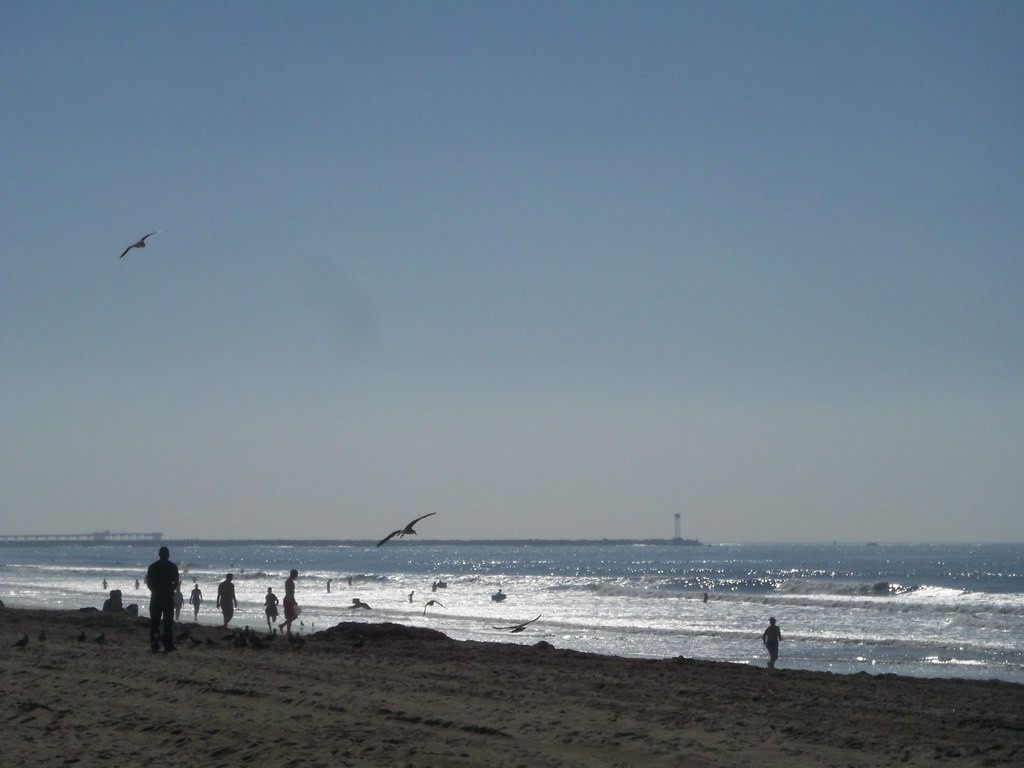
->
[146,547,179,652]
[216,573,237,627]
[763,617,782,669]
[102,576,203,619]
[264,587,279,633]
[278,569,298,636]
[326,576,502,603]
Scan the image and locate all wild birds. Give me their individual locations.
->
[119,232,158,259]
[492,614,541,633]
[377,512,436,548]
[348,597,372,615]
[423,600,445,616]
